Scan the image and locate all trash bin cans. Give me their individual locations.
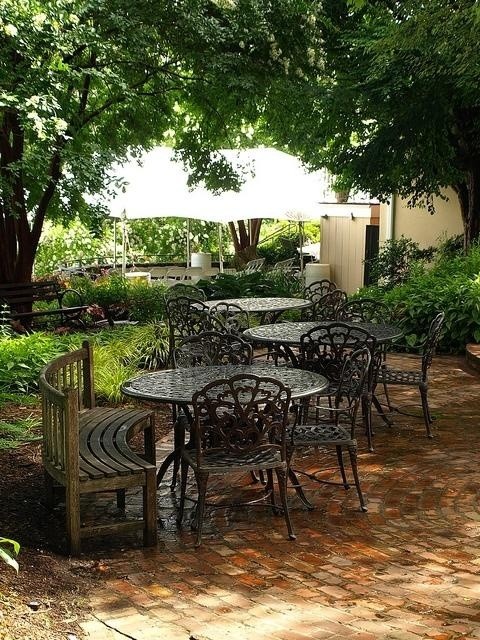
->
[191,253,212,282]
[306,263,331,300]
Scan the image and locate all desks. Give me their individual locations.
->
[243,322,405,437]
[189,297,314,361]
[119,363,330,547]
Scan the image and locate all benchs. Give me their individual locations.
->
[0,281,89,336]
[39,341,157,548]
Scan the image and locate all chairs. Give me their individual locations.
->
[374,312,444,439]
[168,329,257,450]
[297,322,376,455]
[164,282,208,304]
[311,289,351,323]
[173,371,300,542]
[331,298,394,415]
[299,281,337,321]
[272,347,371,514]
[164,294,209,340]
[207,302,291,374]
[58,258,301,285]
